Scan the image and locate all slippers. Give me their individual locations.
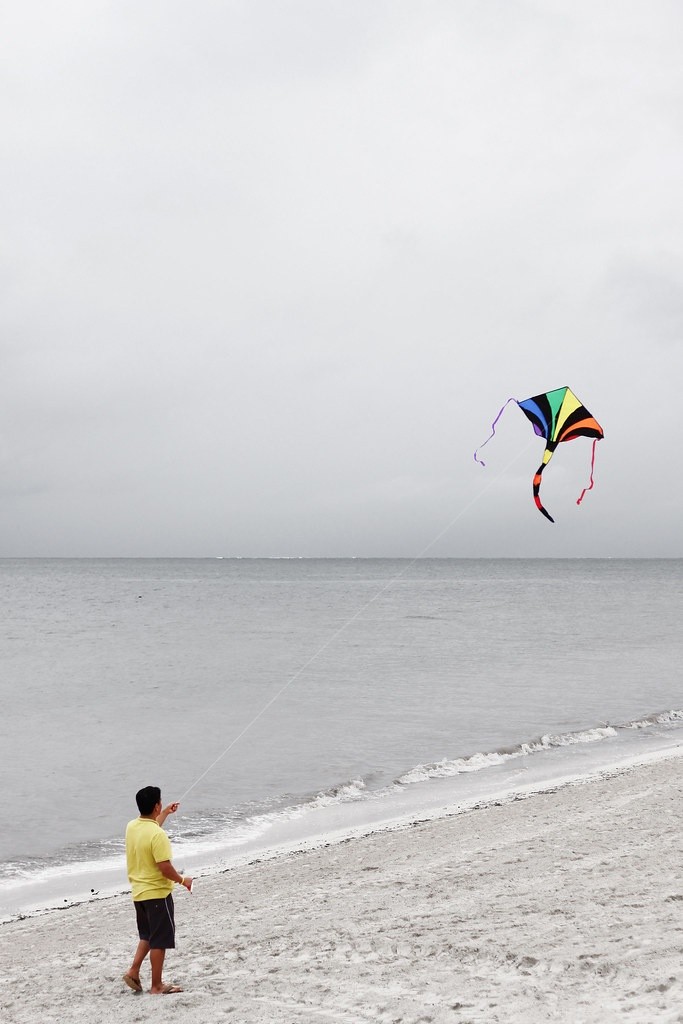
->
[123,974,142,991]
[150,982,184,994]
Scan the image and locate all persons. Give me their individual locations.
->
[123,786,194,994]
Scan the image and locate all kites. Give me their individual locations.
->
[478,380,606,523]
